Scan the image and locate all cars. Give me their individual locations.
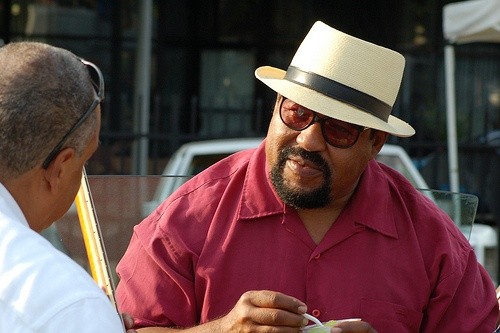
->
[144,137,444,222]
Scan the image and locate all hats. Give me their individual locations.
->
[255,20,416,137]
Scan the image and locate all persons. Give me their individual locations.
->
[114,18,500,333]
[0,41,126,333]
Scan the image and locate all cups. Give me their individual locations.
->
[303,318,362,333]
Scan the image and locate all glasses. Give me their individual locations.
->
[41,57,105,169]
[279,95,374,148]
